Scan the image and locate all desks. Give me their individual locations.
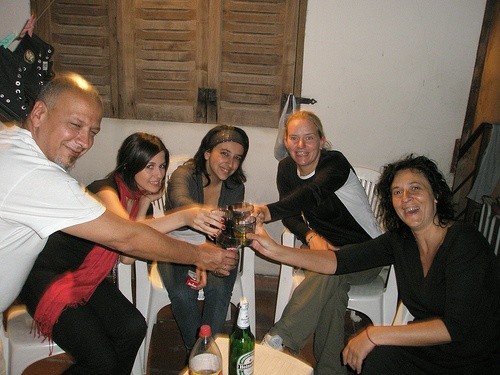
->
[178,335,315,375]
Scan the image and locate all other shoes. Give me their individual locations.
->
[260,333,285,351]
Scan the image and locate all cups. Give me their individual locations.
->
[211,226,243,277]
[211,205,234,238]
[233,216,256,246]
[231,202,254,218]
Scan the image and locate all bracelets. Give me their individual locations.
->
[305,232,320,245]
[364,328,378,348]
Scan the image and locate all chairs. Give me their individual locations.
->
[7,262,131,374]
[136,161,256,339]
[274,167,399,327]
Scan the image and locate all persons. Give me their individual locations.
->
[25,131,226,374]
[245,110,383,375]
[246,151,500,374]
[0,72,238,375]
[157,123,250,355]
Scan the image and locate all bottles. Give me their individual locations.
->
[189,325,223,375]
[228,300,256,375]
